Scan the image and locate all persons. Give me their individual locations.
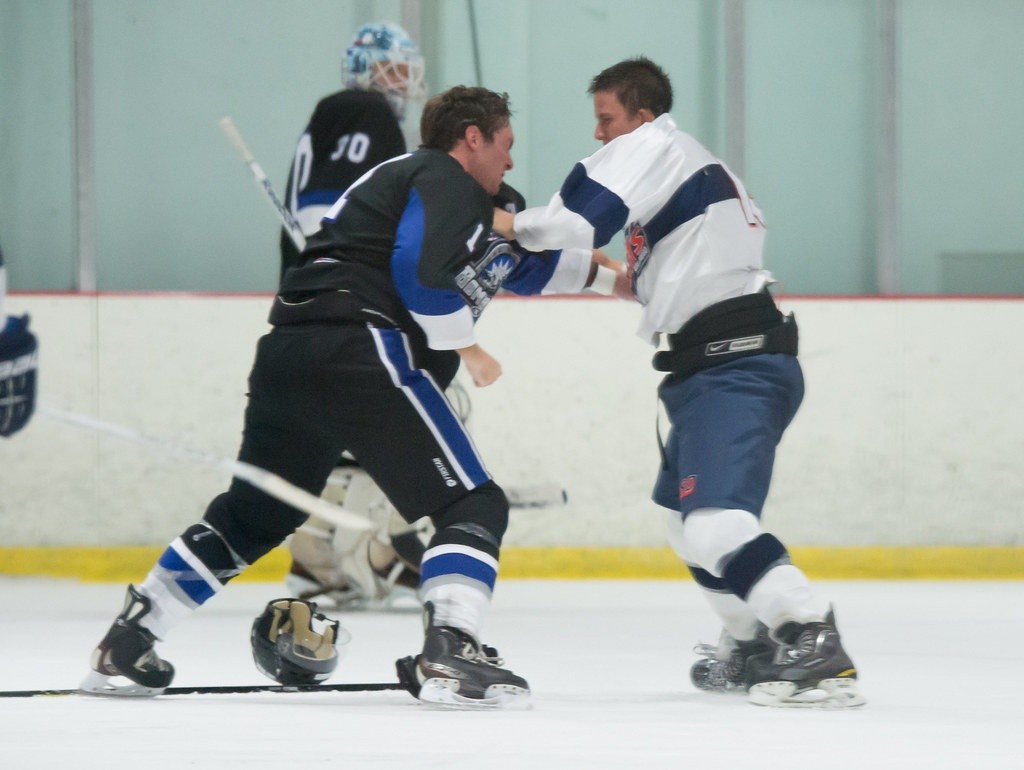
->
[488,58,866,711]
[0,247,40,436]
[79,81,634,707]
[279,19,527,614]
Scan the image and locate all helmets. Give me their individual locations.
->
[248,596,340,685]
[341,21,424,123]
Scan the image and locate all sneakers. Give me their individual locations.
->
[83,584,175,696]
[690,618,778,694]
[745,602,867,708]
[366,537,433,610]
[286,561,366,609]
[416,600,532,711]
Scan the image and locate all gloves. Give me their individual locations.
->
[1,314,37,434]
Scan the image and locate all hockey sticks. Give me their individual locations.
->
[216,114,569,508]
[31,403,431,535]
